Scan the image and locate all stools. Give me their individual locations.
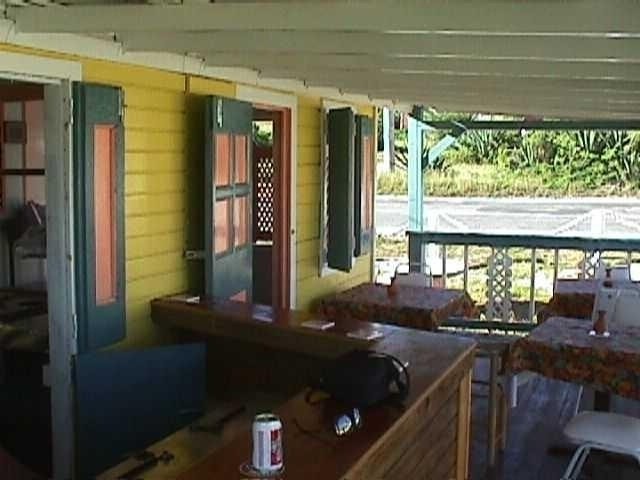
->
[442,333,516,468]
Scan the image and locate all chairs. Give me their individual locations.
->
[591,289,639,331]
[599,257,630,284]
[394,262,433,290]
[559,410,640,479]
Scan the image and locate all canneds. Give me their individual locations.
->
[251,414,285,471]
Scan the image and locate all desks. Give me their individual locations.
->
[537,279,640,326]
[318,281,479,333]
[499,317,640,463]
